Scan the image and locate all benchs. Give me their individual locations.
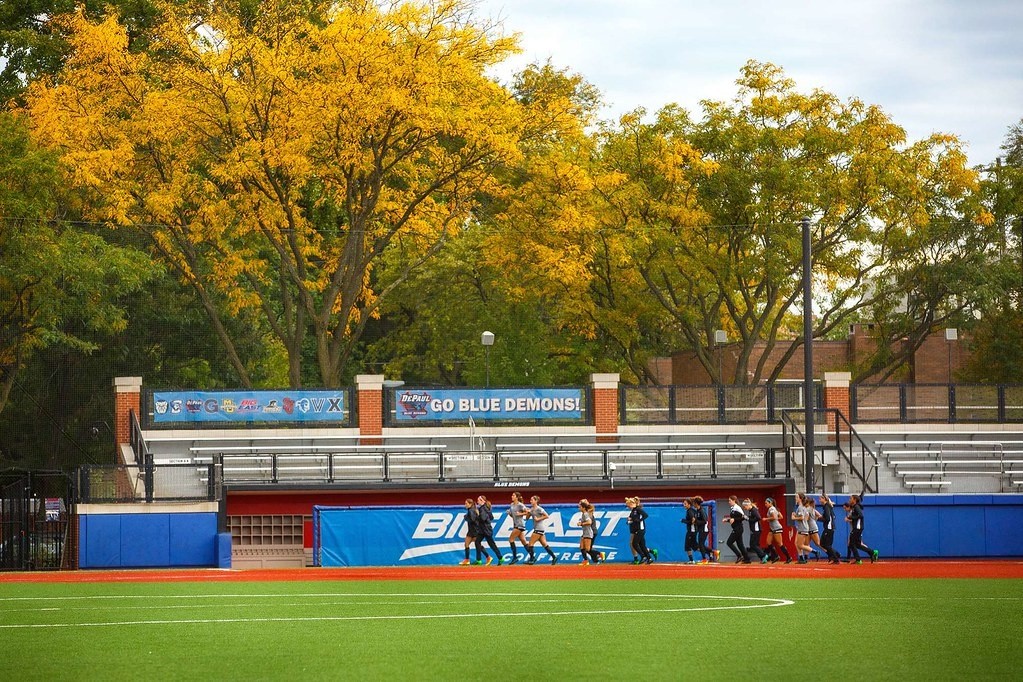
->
[188,440,1023,492]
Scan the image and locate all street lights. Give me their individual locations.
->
[480,328,497,424]
[715,329,729,383]
[945,328,959,381]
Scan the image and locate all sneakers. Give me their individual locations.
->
[551,557,558,565]
[600,551,605,563]
[525,557,534,564]
[629,559,640,565]
[497,558,504,566]
[644,558,653,565]
[485,556,493,566]
[509,557,518,565]
[459,559,470,565]
[471,560,482,566]
[638,556,647,564]
[653,549,658,561]
[686,548,879,567]
[578,560,589,566]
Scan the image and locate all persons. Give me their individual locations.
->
[526,496,558,565]
[626,498,654,565]
[848,495,878,564]
[680,498,713,564]
[791,492,820,564]
[722,495,751,564]
[804,497,828,563]
[577,503,605,566]
[691,496,720,565]
[841,503,878,563]
[473,495,504,566]
[506,491,536,565]
[762,498,792,564]
[819,494,841,564]
[742,498,769,564]
[626,496,657,565]
[462,498,493,566]
[580,498,600,566]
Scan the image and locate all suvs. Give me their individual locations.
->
[0,467,74,545]
